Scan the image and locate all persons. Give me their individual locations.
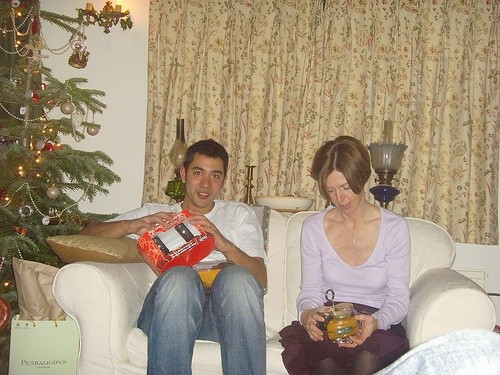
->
[280,135,411,375]
[78,139,268,375]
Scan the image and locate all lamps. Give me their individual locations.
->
[366,119,408,208]
[165,119,188,202]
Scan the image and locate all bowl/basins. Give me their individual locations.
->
[254,196,314,213]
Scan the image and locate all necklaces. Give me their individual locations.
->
[339,203,371,243]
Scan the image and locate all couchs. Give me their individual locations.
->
[53,204,497,375]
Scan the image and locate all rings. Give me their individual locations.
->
[209,223,212,228]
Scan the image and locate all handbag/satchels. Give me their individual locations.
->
[7,314,81,374]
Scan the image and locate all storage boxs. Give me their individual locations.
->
[136,209,215,277]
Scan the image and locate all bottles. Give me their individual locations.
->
[316,302,360,343]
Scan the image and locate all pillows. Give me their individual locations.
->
[46,235,145,263]
